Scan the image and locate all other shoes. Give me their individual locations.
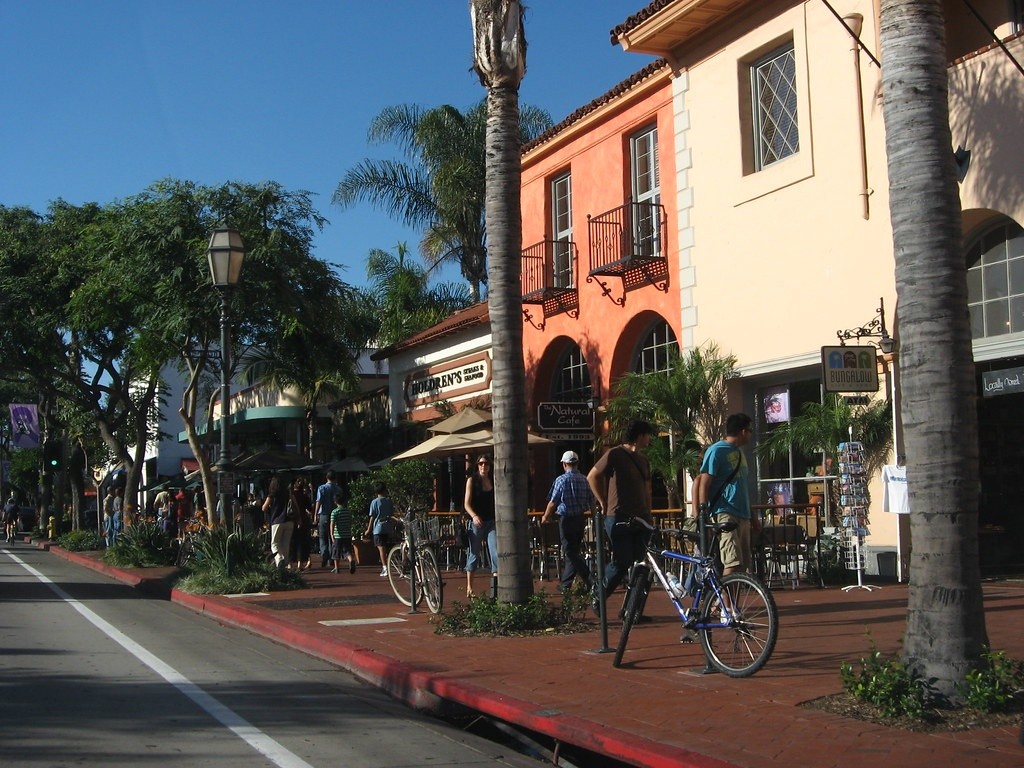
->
[380,568,387,577]
[592,585,601,618]
[721,608,754,628]
[330,567,339,573]
[297,566,304,571]
[304,564,312,571]
[556,585,571,593]
[618,610,653,622]
[330,559,334,565]
[350,560,357,574]
[710,607,723,618]
[322,553,329,567]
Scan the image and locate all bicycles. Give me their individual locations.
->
[8,522,15,546]
[175,518,206,568]
[387,515,445,615]
[613,510,780,678]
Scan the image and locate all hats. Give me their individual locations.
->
[560,450,579,463]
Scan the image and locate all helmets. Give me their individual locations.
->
[8,498,15,504]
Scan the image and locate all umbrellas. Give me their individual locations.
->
[146,445,443,493]
[427,406,494,434]
[390,421,555,484]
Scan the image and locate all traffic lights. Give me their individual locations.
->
[49,443,59,469]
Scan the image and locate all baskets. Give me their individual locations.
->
[404,515,440,548]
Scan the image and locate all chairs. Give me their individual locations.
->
[529,516,827,587]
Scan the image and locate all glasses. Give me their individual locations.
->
[476,461,491,466]
[738,427,756,434]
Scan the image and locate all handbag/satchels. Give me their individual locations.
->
[286,488,300,518]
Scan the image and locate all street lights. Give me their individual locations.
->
[205,209,246,535]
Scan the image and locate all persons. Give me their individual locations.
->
[586,419,653,623]
[364,482,394,577]
[2,498,19,542]
[102,469,360,574]
[463,455,497,599]
[541,450,592,594]
[692,412,754,628]
